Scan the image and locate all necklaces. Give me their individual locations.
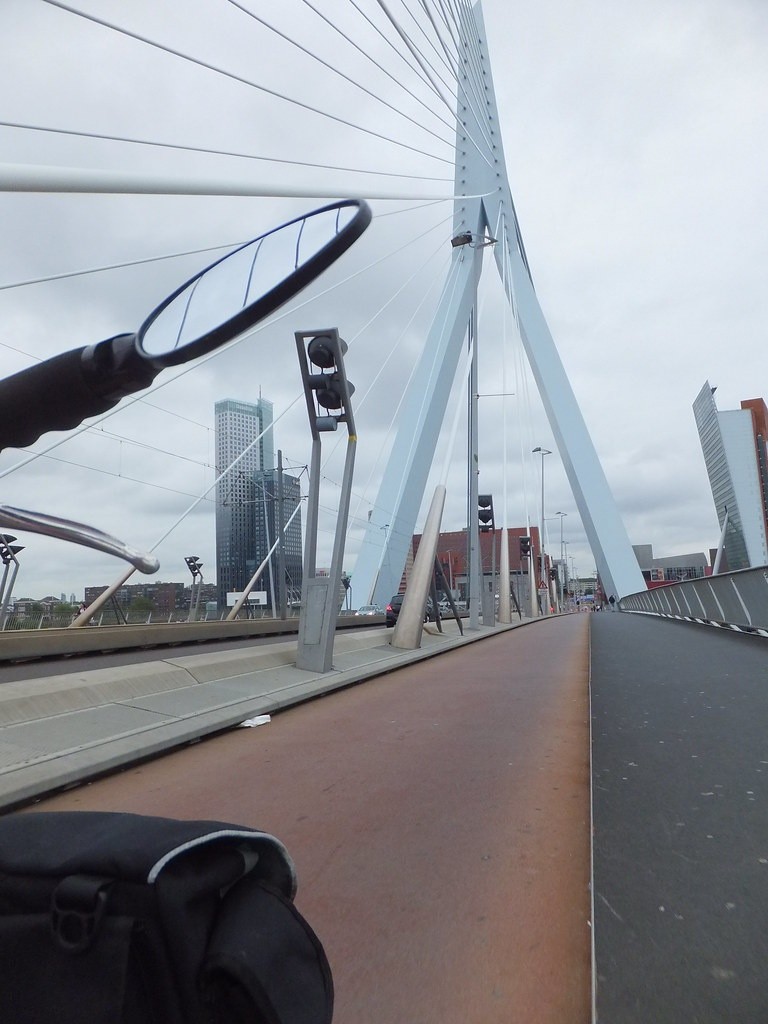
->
[609,595,615,612]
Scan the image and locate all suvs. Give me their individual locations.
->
[386,594,442,626]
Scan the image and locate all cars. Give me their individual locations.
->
[476,594,514,615]
[435,596,470,620]
[354,605,385,615]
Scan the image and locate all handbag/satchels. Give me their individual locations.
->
[0,812,334,1024]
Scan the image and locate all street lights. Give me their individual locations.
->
[380,524,394,568]
[294,326,355,671]
[183,556,204,620]
[517,536,533,617]
[532,446,553,615]
[555,512,567,613]
[563,540,570,565]
[548,567,559,614]
[476,494,495,626]
[592,571,599,602]
[341,577,353,609]
[573,567,579,583]
[569,557,576,606]
[447,549,455,590]
[1,531,25,630]
